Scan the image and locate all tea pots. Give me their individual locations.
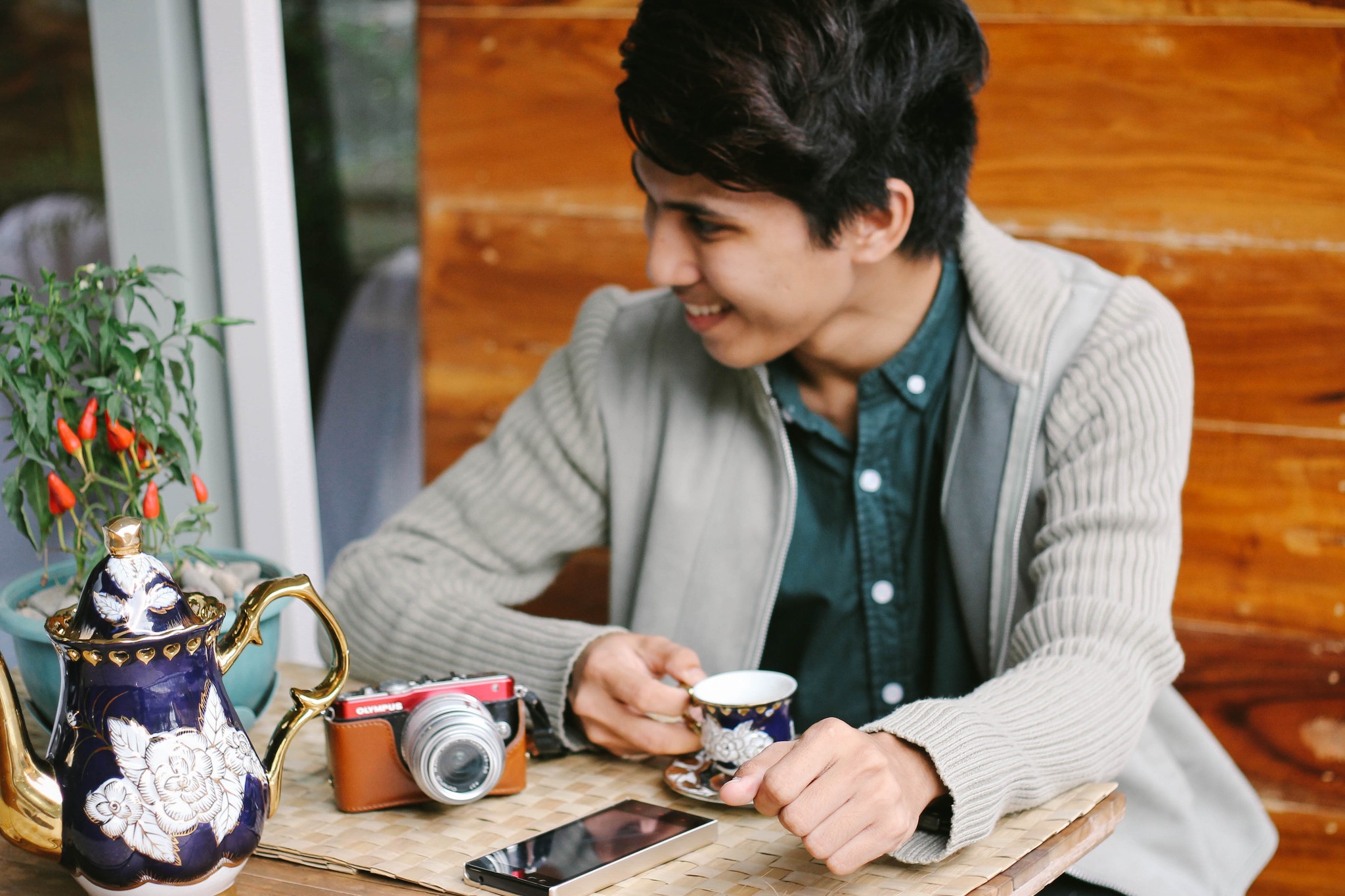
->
[0,516,350,896]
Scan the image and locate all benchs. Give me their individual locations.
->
[405,0,1345,896]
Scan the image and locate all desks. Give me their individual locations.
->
[0,655,1130,896]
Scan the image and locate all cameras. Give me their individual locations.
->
[322,674,527,813]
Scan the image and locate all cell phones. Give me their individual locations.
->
[465,799,719,896]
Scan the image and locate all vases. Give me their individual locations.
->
[0,546,296,730]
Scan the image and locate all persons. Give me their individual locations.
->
[318,0,1277,896]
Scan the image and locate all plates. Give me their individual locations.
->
[665,748,756,805]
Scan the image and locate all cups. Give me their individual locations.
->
[678,670,797,776]
